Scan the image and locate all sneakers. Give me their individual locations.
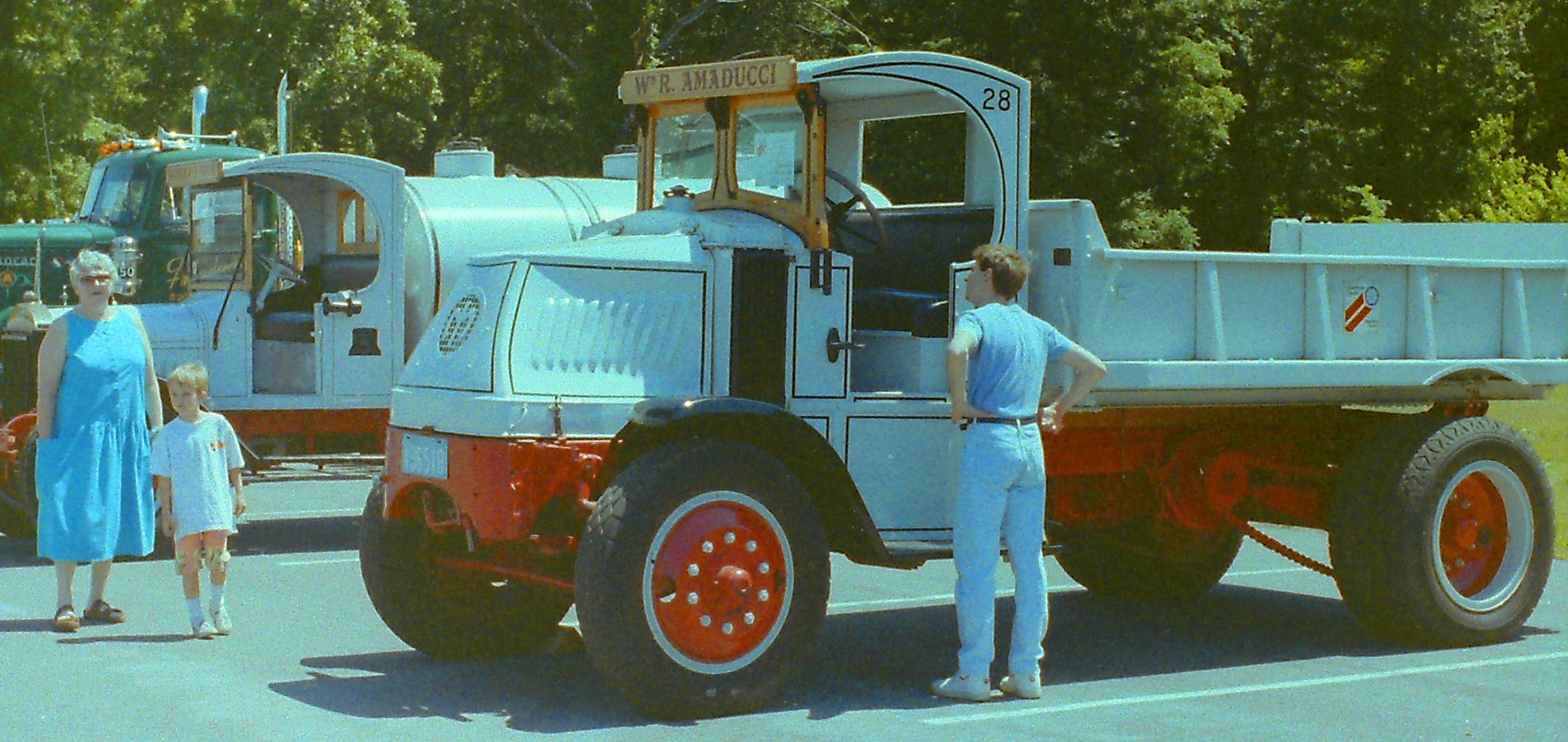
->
[930,672,991,701]
[210,602,232,634]
[193,620,217,639]
[999,674,1041,699]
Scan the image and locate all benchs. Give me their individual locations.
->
[837,204,996,336]
[256,253,380,341]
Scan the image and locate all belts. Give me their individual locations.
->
[960,416,1038,430]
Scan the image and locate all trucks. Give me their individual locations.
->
[0,144,900,553]
[1,74,307,306]
[341,48,1568,721]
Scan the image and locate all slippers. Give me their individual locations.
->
[84,599,125,623]
[54,604,80,632]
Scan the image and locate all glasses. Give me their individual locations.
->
[80,274,113,286]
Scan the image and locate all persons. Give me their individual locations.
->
[930,245,1107,703]
[149,362,247,641]
[34,250,163,629]
